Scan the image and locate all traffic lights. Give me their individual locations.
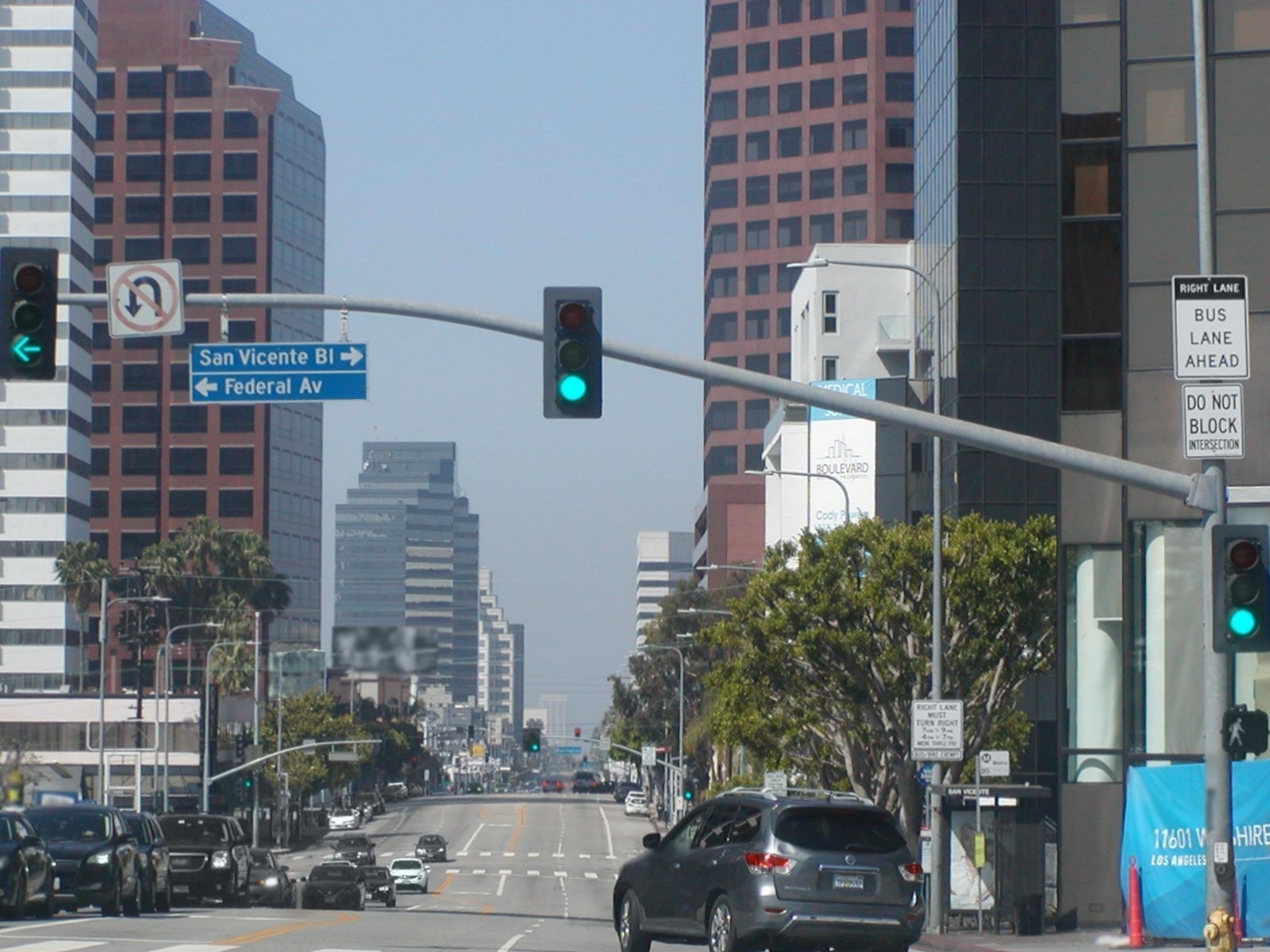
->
[0,246,59,380]
[542,286,602,420]
[235,777,243,802]
[236,734,244,757]
[682,778,694,801]
[1220,708,1269,756]
[584,756,588,766]
[241,771,253,800]
[1211,523,1270,654]
[574,728,581,739]
[523,728,540,752]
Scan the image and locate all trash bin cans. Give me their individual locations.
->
[1016,893,1043,937]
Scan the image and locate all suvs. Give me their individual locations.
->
[16,805,145,917]
[119,808,172,914]
[147,814,253,911]
[612,786,927,952]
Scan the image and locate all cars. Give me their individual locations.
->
[238,780,457,912]
[0,809,60,919]
[459,768,652,819]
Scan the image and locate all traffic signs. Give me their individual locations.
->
[187,342,367,373]
[188,370,367,405]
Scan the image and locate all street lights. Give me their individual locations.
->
[274,649,328,846]
[163,622,224,811]
[743,468,849,522]
[202,640,263,812]
[98,596,173,801]
[785,256,950,936]
[637,643,684,767]
